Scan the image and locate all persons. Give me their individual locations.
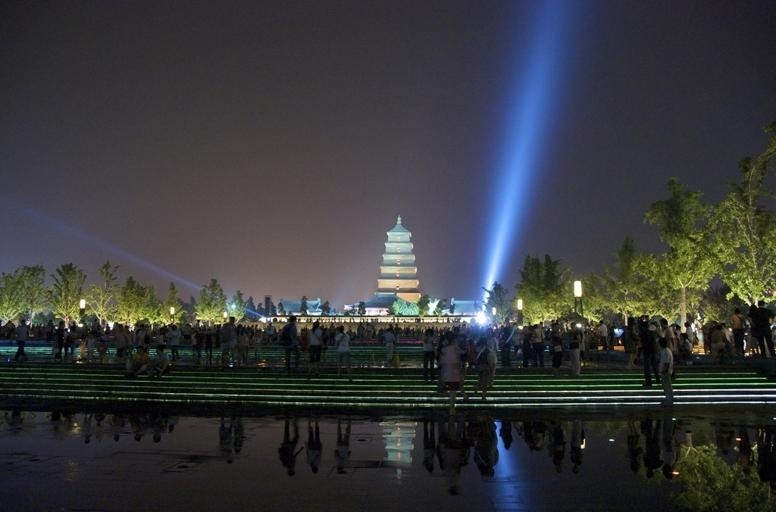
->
[4,413,179,443]
[703,300,775,366]
[626,419,775,488]
[218,416,351,477]
[421,415,585,478]
[397,314,694,410]
[2,315,397,380]
